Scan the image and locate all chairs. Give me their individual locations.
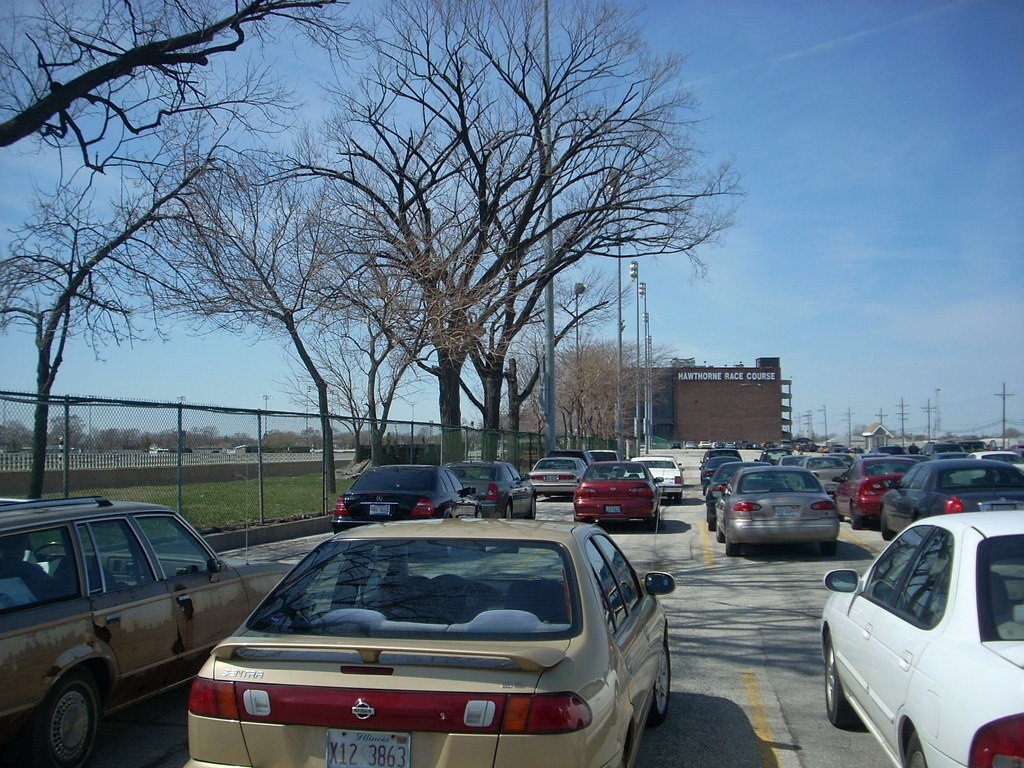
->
[282,571,569,635]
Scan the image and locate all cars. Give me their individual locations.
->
[754,447,811,481]
[880,458,1024,543]
[714,466,841,558]
[699,449,742,498]
[869,440,1024,474]
[442,461,538,519]
[702,460,775,533]
[670,439,864,454]
[329,464,484,535]
[820,509,1023,768]
[831,456,920,530]
[572,461,664,532]
[624,455,685,504]
[527,449,621,499]
[799,457,850,501]
[182,519,676,768]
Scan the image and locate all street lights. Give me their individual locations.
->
[639,280,652,455]
[629,260,640,457]
[572,283,587,362]
[606,168,626,461]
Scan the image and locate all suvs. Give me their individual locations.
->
[0,494,298,768]
